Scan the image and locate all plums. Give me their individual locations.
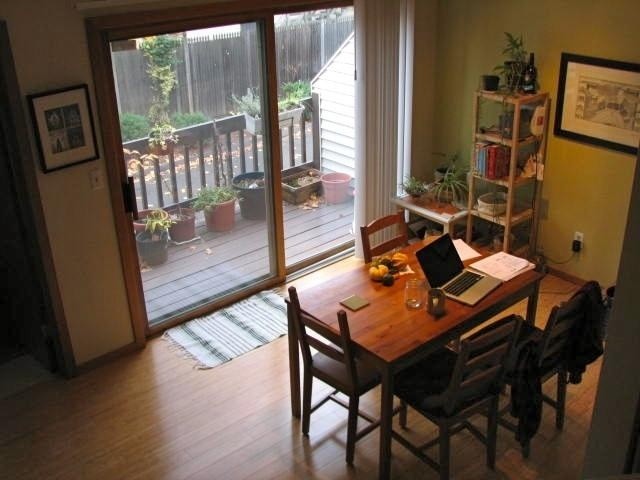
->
[389,268,398,274]
[381,258,392,268]
[382,273,394,285]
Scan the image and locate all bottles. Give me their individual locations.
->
[528,52,536,83]
[522,65,534,94]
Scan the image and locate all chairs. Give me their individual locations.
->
[391,315,523,479]
[288,284,408,468]
[478,279,601,461]
[359,209,409,264]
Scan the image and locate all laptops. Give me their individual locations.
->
[414,232,503,308]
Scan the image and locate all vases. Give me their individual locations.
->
[167,207,197,243]
[320,173,353,206]
[231,170,267,221]
[132,208,169,232]
[483,75,500,92]
[281,167,326,204]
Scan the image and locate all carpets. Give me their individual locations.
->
[161,285,289,371]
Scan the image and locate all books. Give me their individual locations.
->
[452,238,481,262]
[468,250,537,283]
[474,142,510,180]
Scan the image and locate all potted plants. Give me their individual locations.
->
[475,182,507,219]
[433,159,471,210]
[434,151,459,177]
[492,30,535,93]
[149,124,179,156]
[191,184,237,233]
[230,88,305,134]
[400,174,431,198]
[135,218,176,267]
[423,223,440,239]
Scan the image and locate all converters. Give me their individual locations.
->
[572,240,580,252]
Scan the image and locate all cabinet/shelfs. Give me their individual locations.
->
[467,89,551,257]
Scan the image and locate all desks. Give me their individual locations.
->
[284,234,547,480]
[391,192,469,240]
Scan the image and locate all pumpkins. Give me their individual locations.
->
[367,264,389,280]
[392,254,409,268]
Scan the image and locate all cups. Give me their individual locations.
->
[405,279,426,309]
[427,289,445,317]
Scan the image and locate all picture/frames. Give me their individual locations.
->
[25,82,101,174]
[552,52,640,157]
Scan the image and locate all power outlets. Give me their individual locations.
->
[573,231,585,249]
